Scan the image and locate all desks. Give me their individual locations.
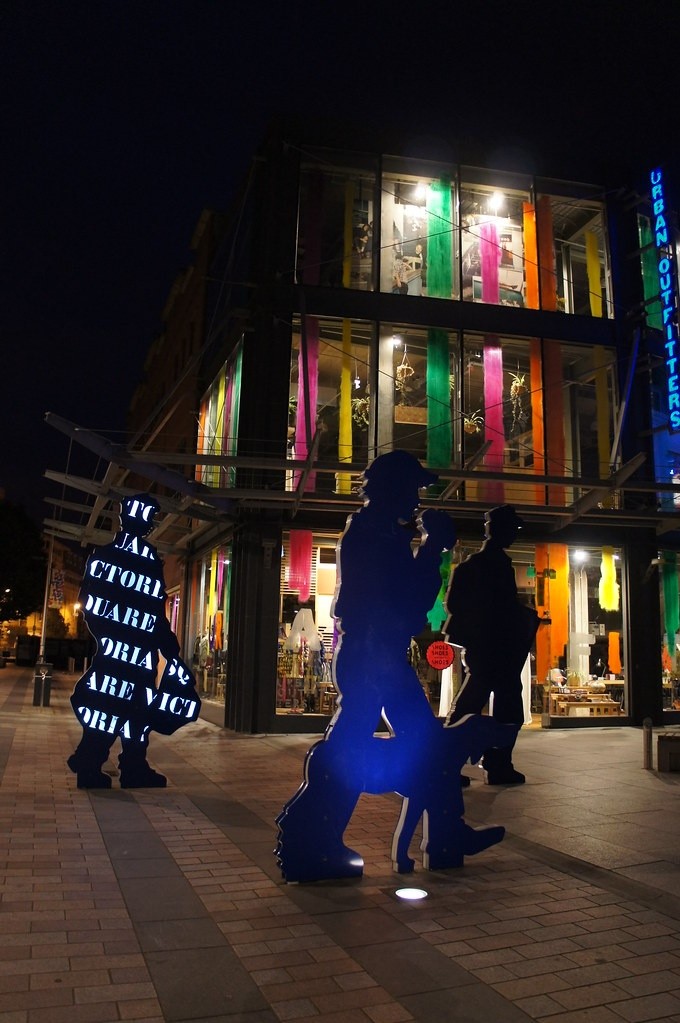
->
[542,685,621,716]
[319,682,339,714]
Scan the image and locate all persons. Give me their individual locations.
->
[301,632,326,713]
[353,222,373,253]
[406,241,422,268]
